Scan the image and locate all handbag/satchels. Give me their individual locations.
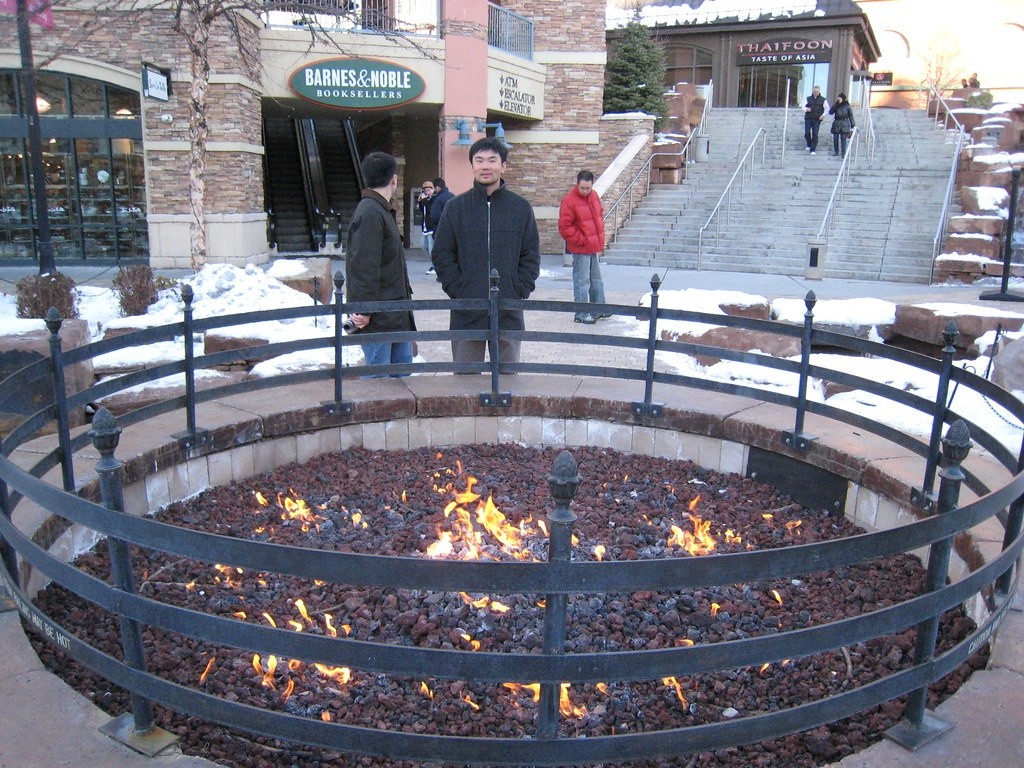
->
[847,130,853,138]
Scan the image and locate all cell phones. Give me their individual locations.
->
[422,191,424,193]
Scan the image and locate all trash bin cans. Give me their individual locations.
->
[695,134,711,164]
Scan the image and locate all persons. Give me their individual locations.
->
[417,181,437,275]
[426,178,456,241]
[802,86,830,156]
[342,151,417,380]
[558,171,611,324]
[829,93,857,158]
[431,137,541,375]
[962,73,980,88]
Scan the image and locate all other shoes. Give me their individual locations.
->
[574,310,596,324]
[425,266,437,274]
[810,152,816,155]
[805,147,810,150]
[591,309,613,319]
[833,154,838,156]
[842,154,845,157]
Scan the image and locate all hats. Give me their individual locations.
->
[423,181,434,189]
[839,93,846,99]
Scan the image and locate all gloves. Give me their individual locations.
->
[835,100,839,106]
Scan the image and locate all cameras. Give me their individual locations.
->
[342,319,359,334]
